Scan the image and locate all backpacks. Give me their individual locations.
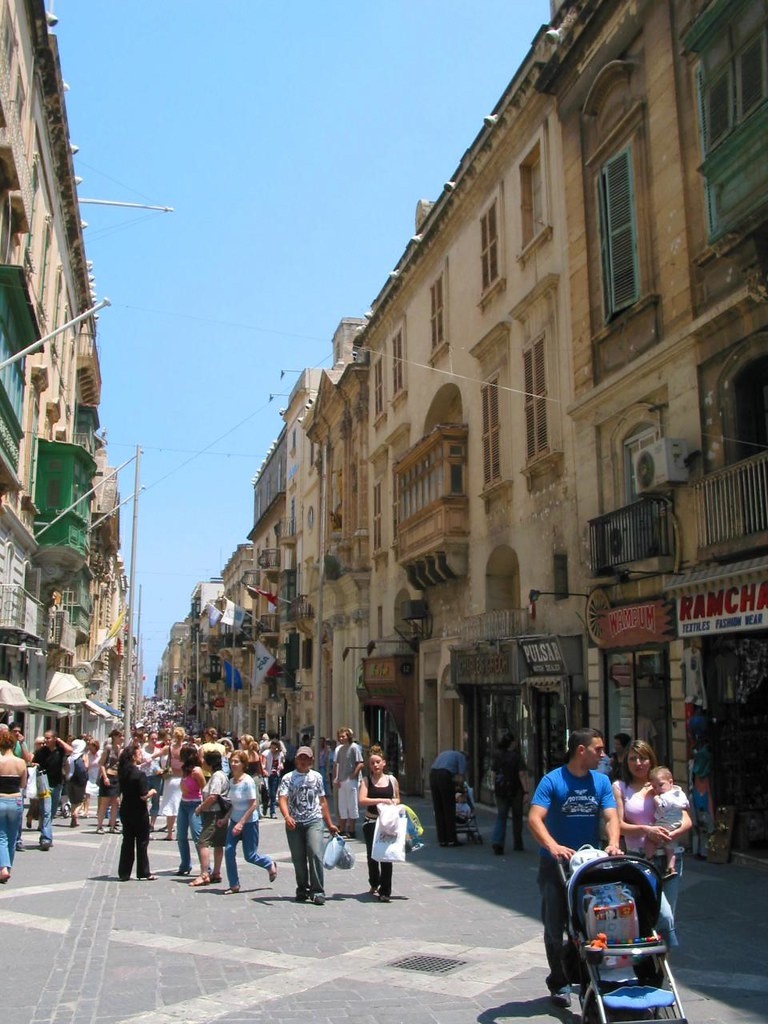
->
[495,760,512,797]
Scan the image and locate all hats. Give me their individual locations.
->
[294,746,313,759]
[72,739,86,753]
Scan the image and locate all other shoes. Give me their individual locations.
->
[295,894,307,903]
[369,881,379,894]
[271,813,277,818]
[440,840,457,847]
[174,867,191,876]
[494,845,502,855]
[551,990,570,1008]
[316,895,325,905]
[665,868,679,881]
[0,814,177,884]
[381,894,390,903]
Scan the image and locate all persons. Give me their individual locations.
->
[0,719,73,884]
[141,725,188,840]
[197,726,287,819]
[188,750,230,885]
[217,749,277,895]
[358,734,530,903]
[609,733,632,782]
[277,746,339,905]
[174,741,211,876]
[611,740,692,985]
[527,724,624,1009]
[319,727,365,838]
[66,727,162,881]
[138,698,202,739]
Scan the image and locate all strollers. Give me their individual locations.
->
[556,844,690,1021]
[456,782,484,845]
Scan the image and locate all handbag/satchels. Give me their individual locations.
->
[76,754,89,785]
[26,765,52,799]
[161,746,173,779]
[322,832,354,869]
[371,803,424,861]
[569,843,614,877]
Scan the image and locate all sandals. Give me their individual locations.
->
[268,862,278,882]
[224,887,240,895]
[210,871,222,883]
[188,872,211,887]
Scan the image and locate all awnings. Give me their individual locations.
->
[82,698,122,720]
[19,696,76,719]
[45,668,87,703]
[0,680,30,725]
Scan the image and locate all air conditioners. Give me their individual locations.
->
[401,600,427,620]
[604,514,651,565]
[634,438,690,495]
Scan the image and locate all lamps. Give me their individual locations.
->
[0,643,48,657]
[444,181,456,192]
[410,234,422,244]
[618,570,684,584]
[545,28,562,44]
[366,637,411,656]
[389,269,399,278]
[528,589,589,601]
[356,325,367,333]
[342,645,376,661]
[44,10,98,320]
[252,370,314,486]
[363,311,372,320]
[483,114,497,127]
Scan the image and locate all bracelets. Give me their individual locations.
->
[525,791,529,794]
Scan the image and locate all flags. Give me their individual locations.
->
[209,605,220,628]
[250,587,277,613]
[220,599,246,629]
[104,610,124,641]
[225,661,243,690]
[252,643,280,688]
